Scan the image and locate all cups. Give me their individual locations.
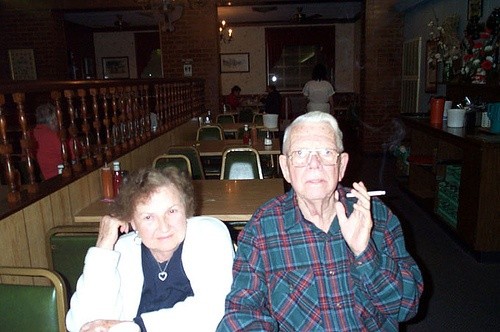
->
[430,98,444,123]
[442,101,453,121]
[487,102,500,133]
[447,109,466,128]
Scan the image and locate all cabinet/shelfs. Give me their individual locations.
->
[396,117,500,254]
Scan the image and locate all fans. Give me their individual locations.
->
[287,7,323,23]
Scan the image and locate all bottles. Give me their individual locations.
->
[101,161,127,200]
[243,124,252,146]
[205,110,212,124]
[70,51,80,81]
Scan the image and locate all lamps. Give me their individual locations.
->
[219,18,233,42]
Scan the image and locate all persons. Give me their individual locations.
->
[216,111,424,332]
[29,103,82,179]
[224,66,336,114]
[65,166,236,332]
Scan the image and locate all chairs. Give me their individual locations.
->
[0,110,277,332]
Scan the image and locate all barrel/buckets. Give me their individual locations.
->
[263,114,279,128]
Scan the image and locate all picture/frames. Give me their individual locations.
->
[424,40,438,94]
[101,56,130,79]
[220,52,250,73]
[467,0,484,20]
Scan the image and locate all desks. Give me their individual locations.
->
[168,137,282,176]
[75,177,284,222]
[222,123,279,132]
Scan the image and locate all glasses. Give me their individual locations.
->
[288,149,340,167]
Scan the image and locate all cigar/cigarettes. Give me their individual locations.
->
[346,191,386,198]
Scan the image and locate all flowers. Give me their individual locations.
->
[424,5,500,86]
